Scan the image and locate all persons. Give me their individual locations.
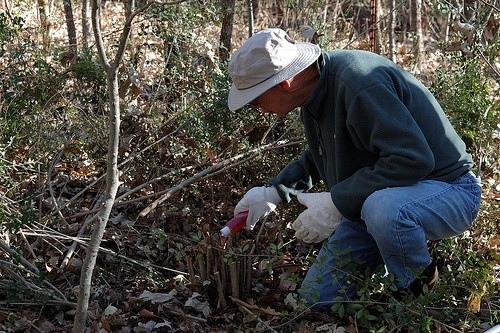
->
[224,27,483,313]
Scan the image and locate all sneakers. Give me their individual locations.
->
[388,260,438,305]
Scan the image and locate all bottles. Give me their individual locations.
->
[221,211,250,237]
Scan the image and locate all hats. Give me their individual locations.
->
[227,28,321,111]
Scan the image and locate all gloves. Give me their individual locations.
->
[292,192,344,244]
[234,184,282,230]
